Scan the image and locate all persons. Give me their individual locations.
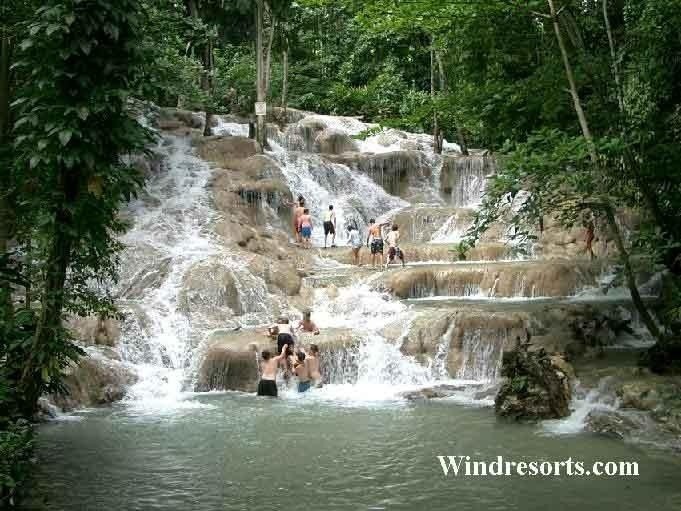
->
[258,310,322,396]
[293,196,404,270]
